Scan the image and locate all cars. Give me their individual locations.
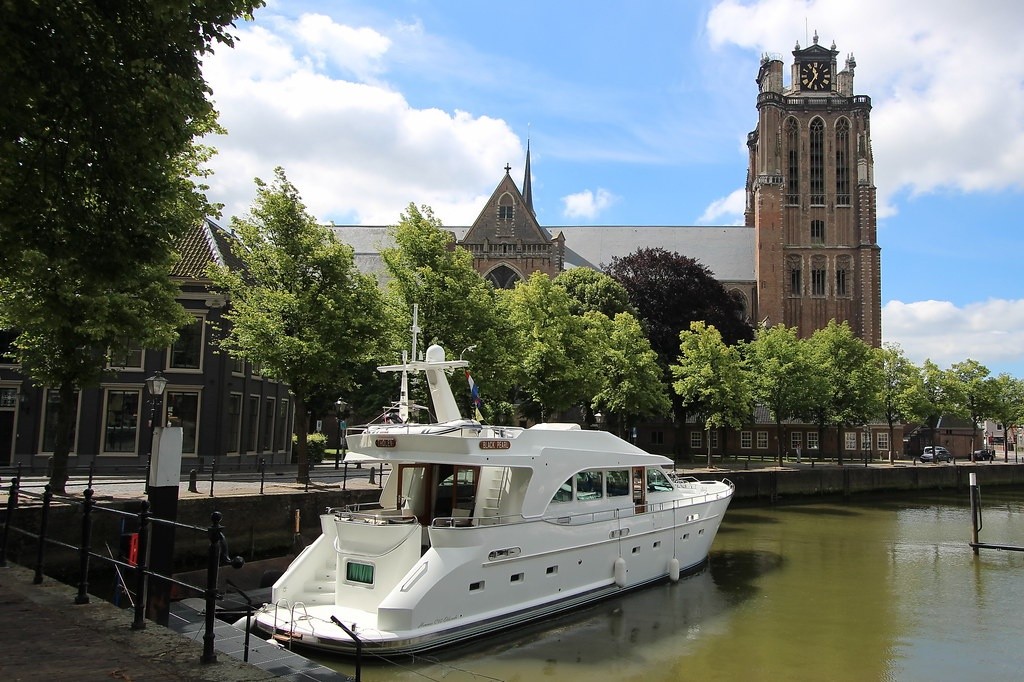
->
[920,449,952,464]
[974,451,990,461]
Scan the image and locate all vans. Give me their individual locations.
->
[924,446,945,455]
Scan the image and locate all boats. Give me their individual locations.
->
[250,303,737,662]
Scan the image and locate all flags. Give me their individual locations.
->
[465,369,481,409]
